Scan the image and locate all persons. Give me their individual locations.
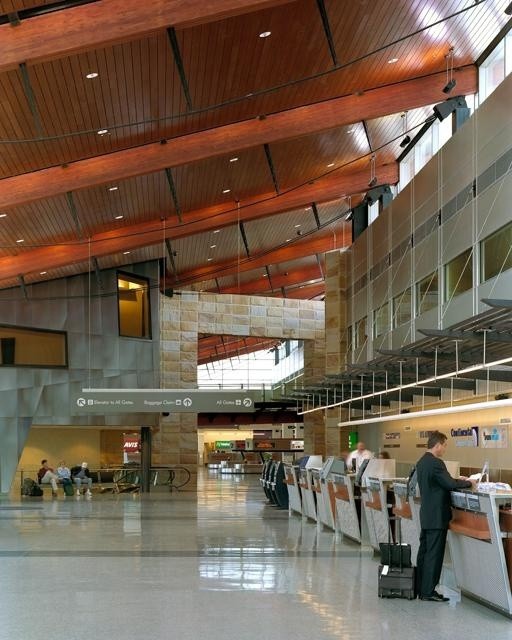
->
[379,451,390,459]
[39,459,64,496]
[71,462,94,496]
[58,460,75,496]
[346,442,375,470]
[413,432,479,603]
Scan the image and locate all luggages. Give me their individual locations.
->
[377,565,418,601]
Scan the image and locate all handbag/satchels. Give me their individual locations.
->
[63,483,74,496]
[30,481,43,496]
[377,540,412,568]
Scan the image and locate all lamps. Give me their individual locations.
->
[345,196,353,222]
[368,152,378,187]
[441,47,457,94]
[400,110,410,148]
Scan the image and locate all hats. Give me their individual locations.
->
[81,461,88,468]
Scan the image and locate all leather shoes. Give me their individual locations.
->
[419,593,450,602]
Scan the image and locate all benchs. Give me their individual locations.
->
[37,472,120,493]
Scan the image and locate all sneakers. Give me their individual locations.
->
[76,488,92,496]
